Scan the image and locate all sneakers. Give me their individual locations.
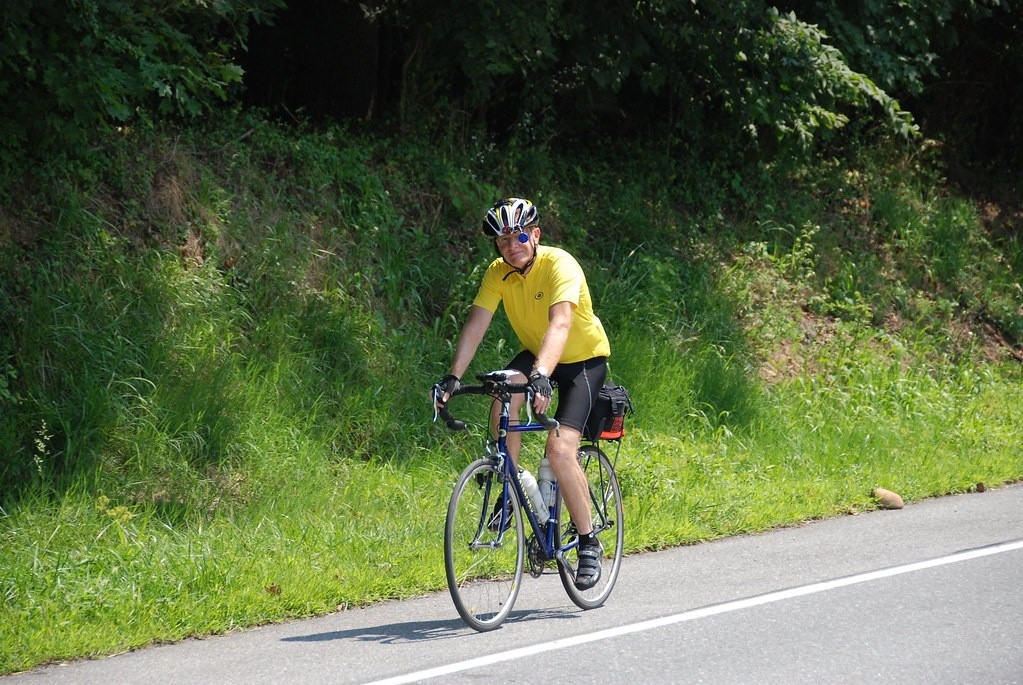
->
[573,538,604,591]
[487,491,514,531]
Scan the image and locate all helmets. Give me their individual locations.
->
[482,198,540,239]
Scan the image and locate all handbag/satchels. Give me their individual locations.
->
[583,382,635,442]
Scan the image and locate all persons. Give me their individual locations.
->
[429,198,610,591]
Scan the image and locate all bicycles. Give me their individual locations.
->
[429,368,626,632]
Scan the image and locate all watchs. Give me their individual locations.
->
[531,366,547,376]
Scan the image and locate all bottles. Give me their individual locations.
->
[518,469,550,524]
[537,454,556,519]
